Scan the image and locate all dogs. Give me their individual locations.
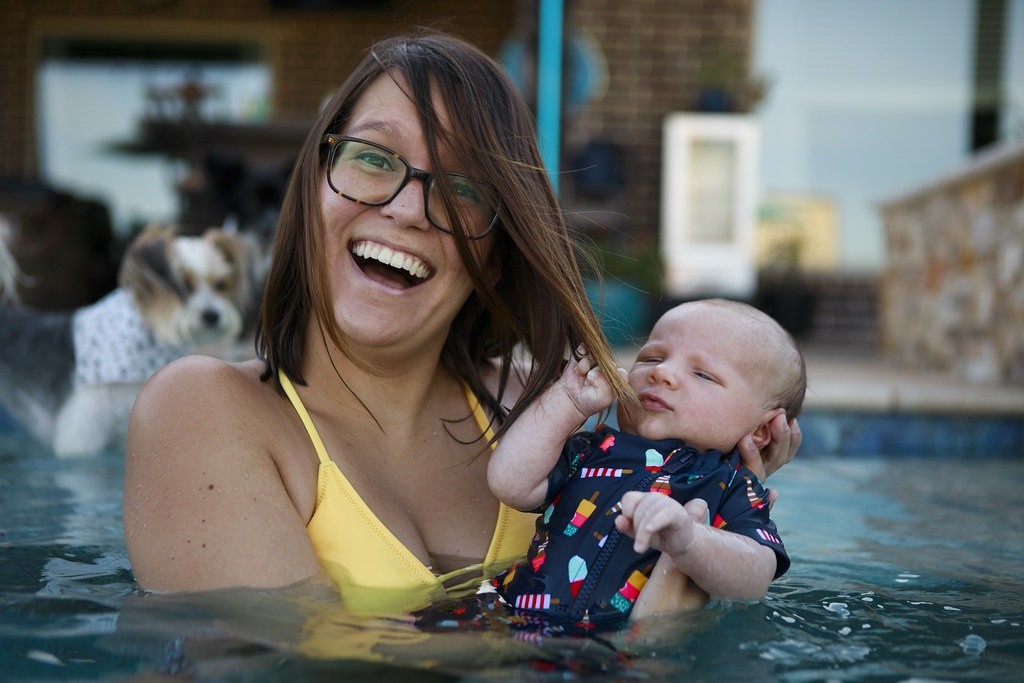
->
[0,223,251,455]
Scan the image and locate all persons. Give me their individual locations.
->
[486,298,807,615]
[743,502,752,503]
[122,33,802,616]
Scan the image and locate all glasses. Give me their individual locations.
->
[320,133,502,241]
